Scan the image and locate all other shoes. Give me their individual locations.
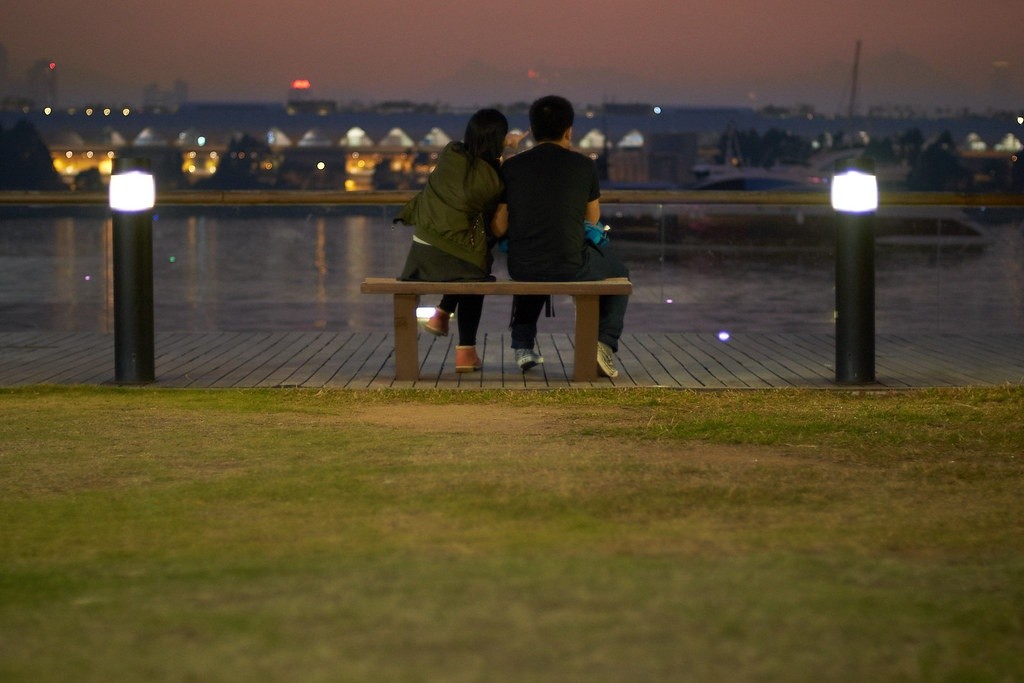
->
[455,345,482,372]
[425,308,448,336]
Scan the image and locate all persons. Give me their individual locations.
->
[392,107,530,373]
[491,94,630,378]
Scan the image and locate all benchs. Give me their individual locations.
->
[360,274,632,384]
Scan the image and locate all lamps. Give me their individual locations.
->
[108,169,157,213]
[831,169,879,213]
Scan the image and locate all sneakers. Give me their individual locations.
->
[597,342,618,378]
[515,348,545,371]
